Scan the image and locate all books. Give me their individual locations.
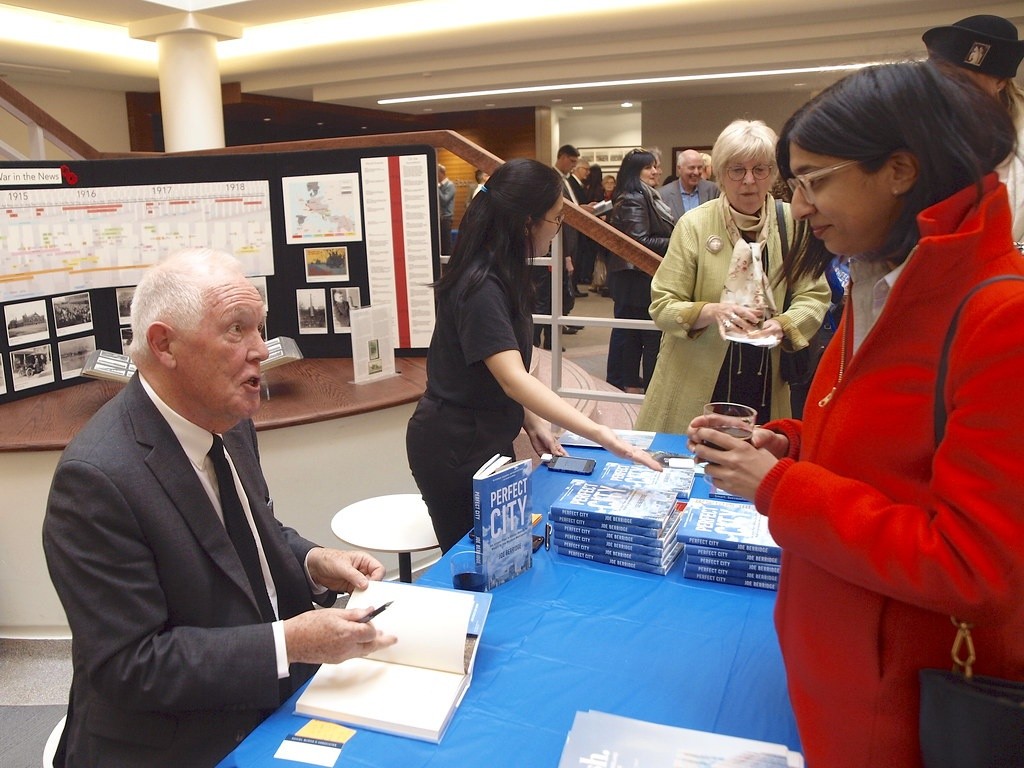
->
[472,454,533,592]
[558,709,805,768]
[557,429,656,451]
[547,461,783,591]
[291,580,493,745]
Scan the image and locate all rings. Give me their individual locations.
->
[729,313,737,323]
[723,320,731,329]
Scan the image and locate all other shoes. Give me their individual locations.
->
[626,376,644,388]
[588,287,597,292]
[562,325,584,334]
[574,290,589,297]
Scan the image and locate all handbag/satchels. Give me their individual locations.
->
[919,665,1024,768]
[778,322,835,392]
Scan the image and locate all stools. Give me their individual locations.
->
[331,494,440,582]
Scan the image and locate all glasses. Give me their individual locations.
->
[787,157,874,205]
[628,148,645,160]
[542,213,566,234]
[726,163,775,182]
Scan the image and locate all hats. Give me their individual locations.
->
[922,14,1024,78]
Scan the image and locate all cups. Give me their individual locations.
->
[451,551,489,592]
[702,402,757,465]
[737,280,766,335]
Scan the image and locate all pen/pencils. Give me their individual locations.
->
[544,522,552,550]
[356,600,393,623]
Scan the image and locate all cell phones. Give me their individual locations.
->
[548,454,597,476]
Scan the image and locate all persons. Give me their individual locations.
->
[686,59,1024,768]
[43,255,398,768]
[406,158,662,555]
[437,164,456,275]
[922,14,1024,256]
[469,119,852,436]
[333,291,351,327]
[33,355,41,372]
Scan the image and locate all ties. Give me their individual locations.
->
[207,432,294,709]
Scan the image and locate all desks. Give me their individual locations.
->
[214,428,796,768]
